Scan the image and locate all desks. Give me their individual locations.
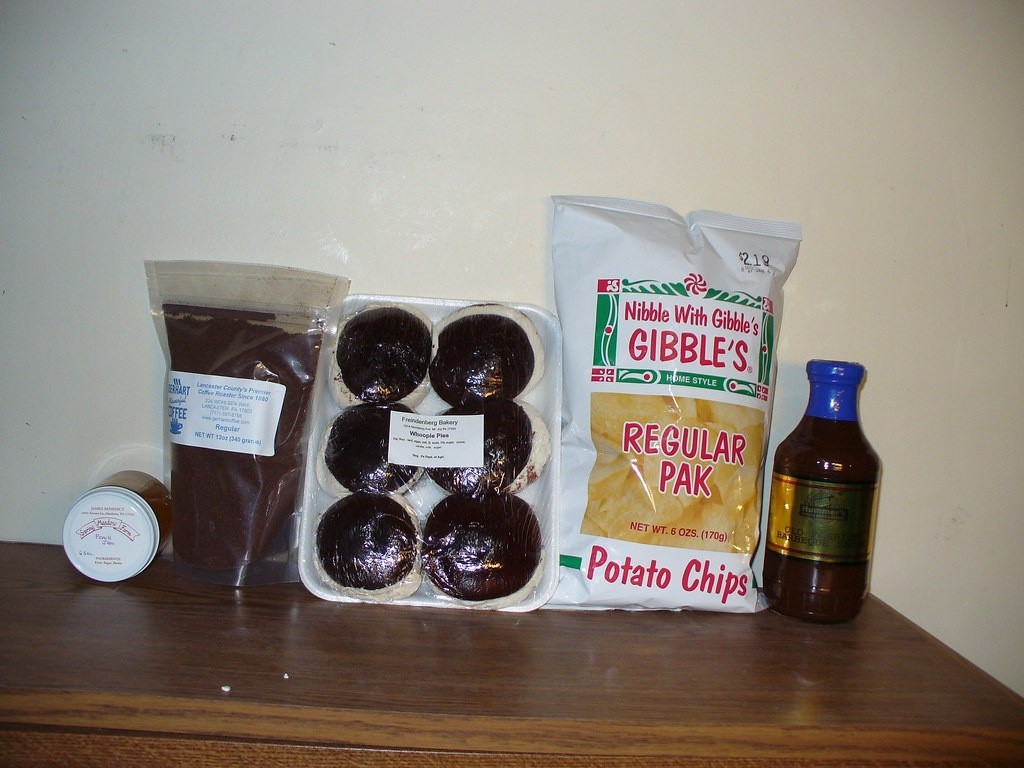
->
[0,542,1024,768]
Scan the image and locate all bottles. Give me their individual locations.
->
[760,360,885,624]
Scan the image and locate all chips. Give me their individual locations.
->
[580,392,763,557]
